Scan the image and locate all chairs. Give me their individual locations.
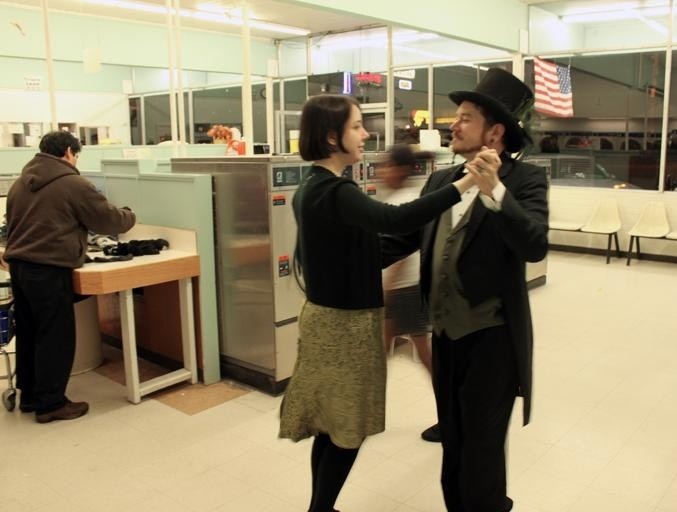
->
[546,190,676,266]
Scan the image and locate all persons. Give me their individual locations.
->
[378,144,436,442]
[381,99,548,512]
[3,131,136,423]
[279,94,502,512]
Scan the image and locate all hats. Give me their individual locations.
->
[448,67,535,147]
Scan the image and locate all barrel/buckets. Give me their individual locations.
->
[67,295,107,378]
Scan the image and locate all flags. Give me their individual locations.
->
[533,55,574,118]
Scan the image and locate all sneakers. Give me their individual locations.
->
[19,392,89,423]
[422,423,442,443]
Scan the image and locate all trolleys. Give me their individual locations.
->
[0,278,17,412]
